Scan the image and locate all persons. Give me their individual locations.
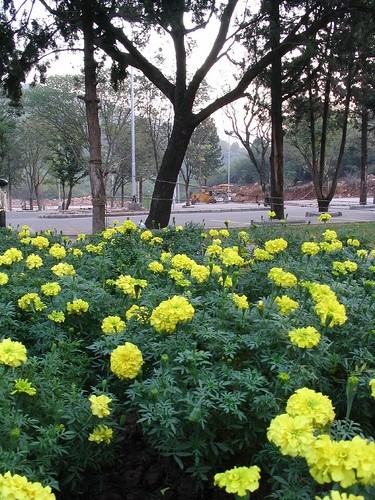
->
[0,178,9,229]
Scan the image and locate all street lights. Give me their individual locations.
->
[224,130,235,189]
[127,21,139,203]
